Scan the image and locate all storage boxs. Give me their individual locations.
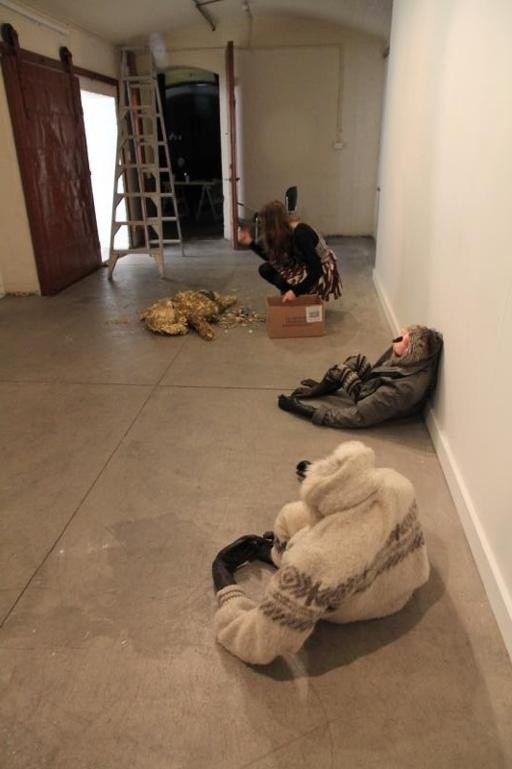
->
[264,296,326,339]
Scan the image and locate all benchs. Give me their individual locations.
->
[162,181,219,223]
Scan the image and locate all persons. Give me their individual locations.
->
[213,438,434,679]
[276,325,440,431]
[238,198,344,303]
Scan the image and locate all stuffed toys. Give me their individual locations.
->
[139,286,239,341]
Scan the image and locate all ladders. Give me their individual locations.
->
[108,43,185,280]
